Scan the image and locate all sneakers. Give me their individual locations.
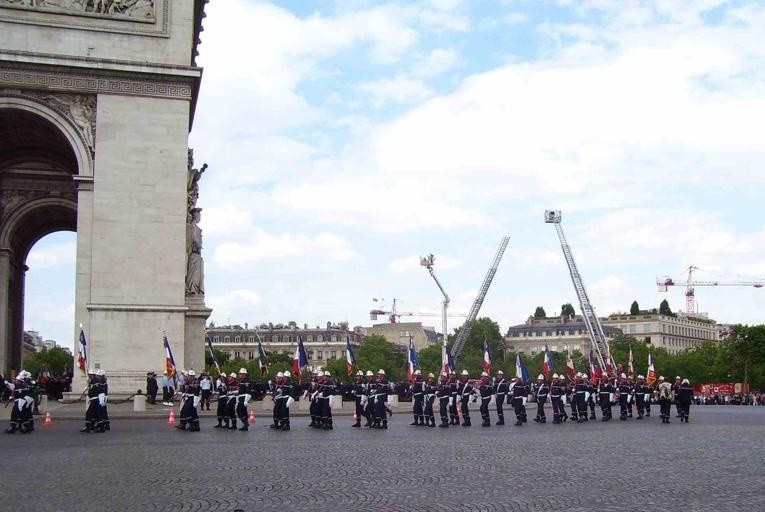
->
[389,409,393,417]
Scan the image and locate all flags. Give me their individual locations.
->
[163,337,176,380]
[293,336,308,380]
[207,337,221,375]
[408,335,656,384]
[258,338,269,375]
[77,330,88,374]
[346,336,355,375]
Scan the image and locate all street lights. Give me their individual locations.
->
[727,374,732,391]
[708,371,713,382]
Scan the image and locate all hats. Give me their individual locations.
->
[377,369,386,376]
[182,371,195,376]
[312,369,330,377]
[228,372,237,379]
[15,371,33,381]
[220,372,227,378]
[412,370,690,385]
[355,369,364,376]
[237,367,248,375]
[88,369,105,376]
[365,370,374,376]
[276,371,291,377]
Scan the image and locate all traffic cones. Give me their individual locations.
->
[352,408,358,422]
[456,405,462,418]
[248,409,257,424]
[43,411,55,426]
[168,410,176,425]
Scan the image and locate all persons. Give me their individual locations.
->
[185,148,208,295]
[41,94,95,153]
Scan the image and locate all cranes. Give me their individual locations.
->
[656,264,765,316]
[367,296,468,326]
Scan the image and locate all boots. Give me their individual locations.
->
[351,417,362,428]
[222,418,230,428]
[271,418,290,431]
[228,419,237,430]
[80,421,111,433]
[309,416,333,430]
[5,420,35,434]
[237,418,249,431]
[176,419,200,431]
[364,417,388,429]
[408,409,690,429]
[214,417,223,428]
